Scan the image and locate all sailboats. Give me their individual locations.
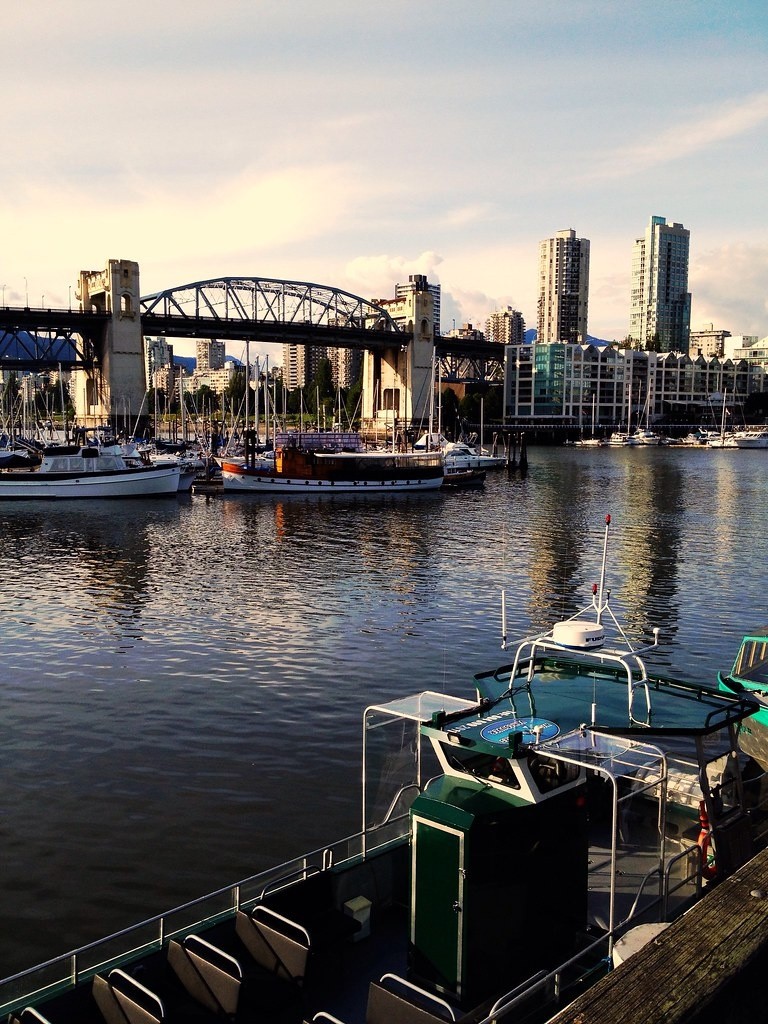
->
[563,378,768,449]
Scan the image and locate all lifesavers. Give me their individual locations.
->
[697,829,718,875]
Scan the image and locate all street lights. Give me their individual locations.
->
[2,285,5,309]
[23,277,28,309]
[41,295,44,310]
[453,319,455,338]
[69,286,72,311]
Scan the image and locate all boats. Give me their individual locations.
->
[0,512,768,1024]
[1,355,509,499]
